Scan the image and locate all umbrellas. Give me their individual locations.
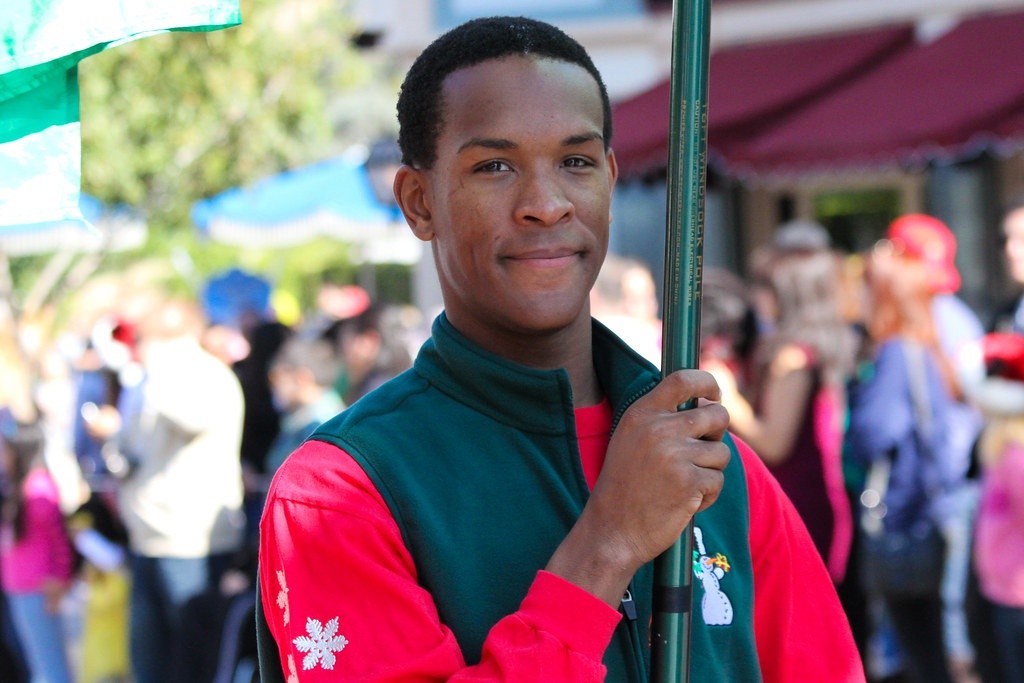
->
[197,155,405,244]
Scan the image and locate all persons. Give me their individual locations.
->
[592,204,1023,683]
[0,284,411,683]
[254,16,864,683]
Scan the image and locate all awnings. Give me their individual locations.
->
[611,13,1024,182]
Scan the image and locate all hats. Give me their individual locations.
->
[886,214,961,293]
[771,219,829,251]
